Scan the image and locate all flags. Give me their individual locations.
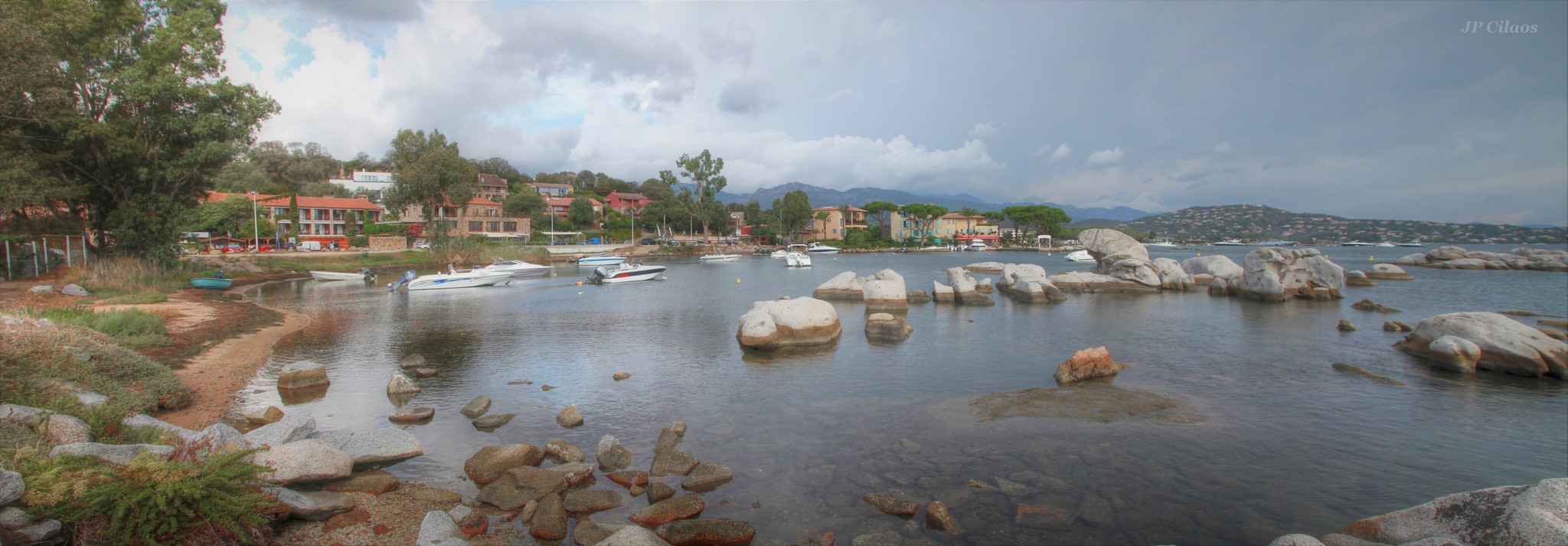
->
[708,230,711,236]
[228,230,232,238]
[681,229,686,236]
[661,230,665,238]
[719,232,723,238]
[692,230,698,236]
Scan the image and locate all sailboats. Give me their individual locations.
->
[771,208,788,258]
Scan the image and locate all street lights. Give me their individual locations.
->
[736,216,738,241]
[549,206,553,245]
[251,191,259,252]
[630,210,633,244]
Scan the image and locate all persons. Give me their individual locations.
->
[956,246,959,251]
[675,238,677,241]
[949,246,951,251]
[330,240,334,251]
[953,247,955,251]
[959,246,962,251]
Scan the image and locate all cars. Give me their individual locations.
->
[171,245,199,255]
[547,238,608,245]
[720,239,738,245]
[623,240,630,244]
[641,238,679,246]
[220,240,341,254]
[416,242,437,248]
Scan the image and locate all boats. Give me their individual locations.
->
[896,247,910,253]
[584,259,667,284]
[469,256,555,277]
[783,244,811,267]
[1340,239,1426,248]
[1212,239,1244,246]
[574,252,628,266]
[916,239,987,251]
[697,246,742,261]
[808,242,842,254]
[188,271,234,290]
[1063,250,1096,261]
[1148,241,1177,247]
[309,267,378,281]
[1257,240,1298,246]
[387,263,515,290]
